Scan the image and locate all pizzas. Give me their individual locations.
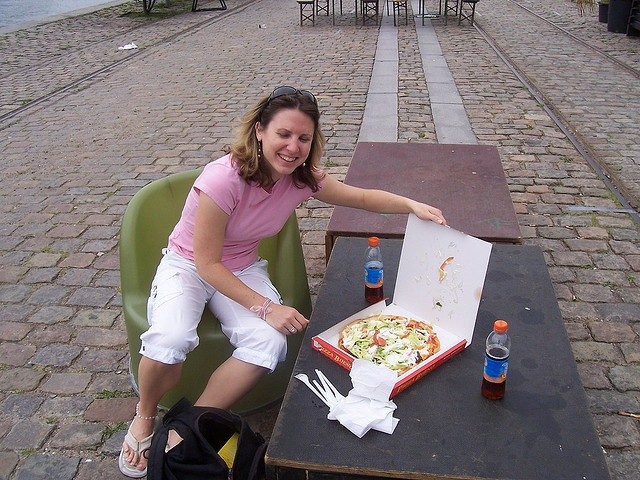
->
[338,314,441,377]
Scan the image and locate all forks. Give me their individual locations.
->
[294,372,330,408]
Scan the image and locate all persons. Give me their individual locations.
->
[118,87,446,478]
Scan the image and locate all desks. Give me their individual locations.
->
[325,142,522,266]
[265,234,611,480]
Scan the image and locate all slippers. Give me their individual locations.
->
[118,416,155,477]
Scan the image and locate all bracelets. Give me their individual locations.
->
[135,402,159,420]
[249,298,273,320]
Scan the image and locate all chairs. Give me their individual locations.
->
[120,168,313,417]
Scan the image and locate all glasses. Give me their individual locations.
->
[267,86,318,107]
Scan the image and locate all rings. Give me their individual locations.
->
[288,327,295,332]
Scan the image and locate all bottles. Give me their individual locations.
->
[480,320,511,400]
[363,237,384,305]
